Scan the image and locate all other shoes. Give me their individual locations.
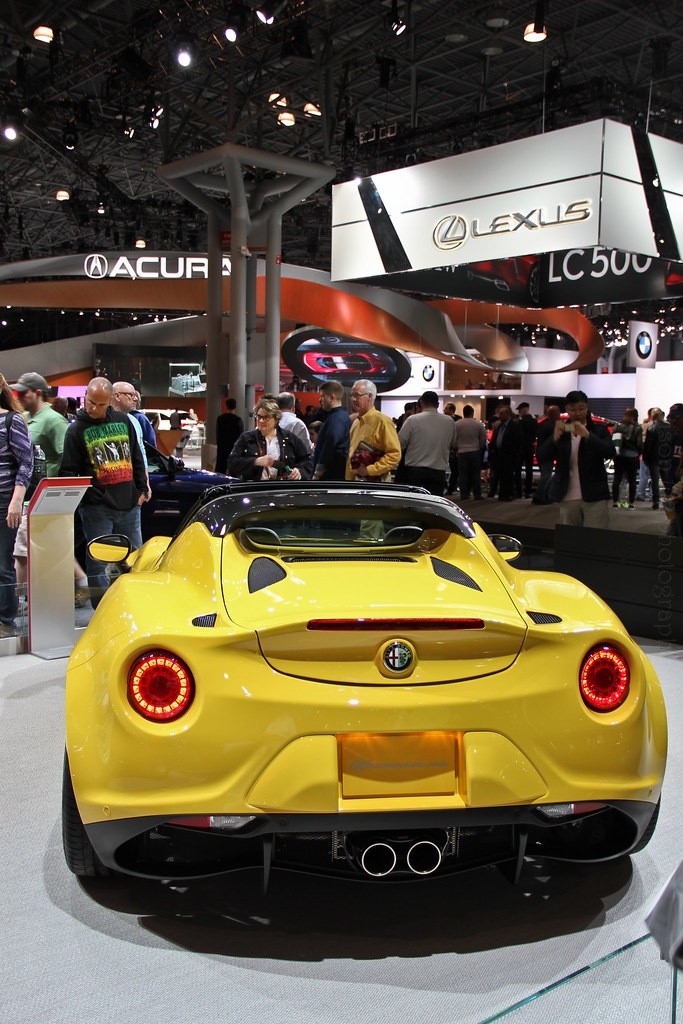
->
[461,495,471,498]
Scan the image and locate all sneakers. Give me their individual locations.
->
[649,498,653,501]
[613,500,623,510]
[623,501,636,511]
[74,588,91,609]
[475,496,485,500]
[0,622,16,637]
[635,496,645,501]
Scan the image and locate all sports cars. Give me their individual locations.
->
[61,481,667,909]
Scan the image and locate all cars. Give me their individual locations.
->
[486,411,623,475]
[137,409,200,433]
[141,439,240,541]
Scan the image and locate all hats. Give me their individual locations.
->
[516,402,529,410]
[9,372,48,392]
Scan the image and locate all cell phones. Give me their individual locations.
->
[562,423,576,431]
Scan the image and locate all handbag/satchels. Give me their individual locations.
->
[611,423,622,455]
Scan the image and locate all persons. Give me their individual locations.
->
[215,398,244,477]
[345,379,400,482]
[170,409,182,430]
[295,398,327,426]
[443,402,683,535]
[536,390,615,529]
[189,409,198,421]
[278,392,313,455]
[397,391,457,498]
[130,391,157,448]
[309,421,324,444]
[11,372,89,614]
[393,402,423,433]
[0,373,33,638]
[110,382,152,503]
[58,377,148,613]
[312,381,351,480]
[228,400,313,481]
[51,397,78,421]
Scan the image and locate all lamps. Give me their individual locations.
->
[2,110,23,139]
[49,184,188,252]
[33,26,53,42]
[384,0,407,36]
[224,7,251,42]
[521,0,549,43]
[264,82,331,131]
[253,0,289,25]
[116,115,135,140]
[174,36,196,68]
[143,86,166,130]
[63,121,78,150]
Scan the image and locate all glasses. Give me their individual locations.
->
[254,413,276,421]
[118,391,138,398]
[352,393,368,398]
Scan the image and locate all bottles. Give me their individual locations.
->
[34,445,45,472]
[286,466,301,480]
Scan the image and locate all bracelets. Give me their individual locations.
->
[313,472,318,477]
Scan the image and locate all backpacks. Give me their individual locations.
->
[6,411,47,501]
